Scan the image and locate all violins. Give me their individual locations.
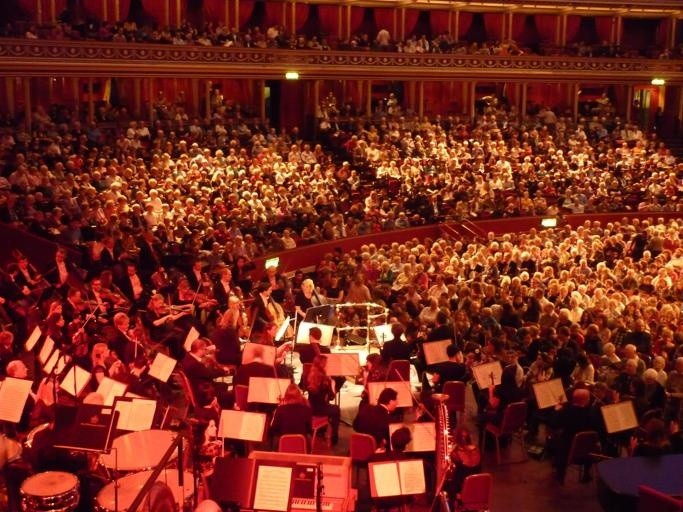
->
[158,302,191,315]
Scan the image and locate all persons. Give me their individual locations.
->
[0,16,682,512]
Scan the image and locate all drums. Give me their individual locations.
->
[17,472,81,512]
[94,429,191,485]
[93,467,198,512]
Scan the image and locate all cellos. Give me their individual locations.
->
[266,292,294,338]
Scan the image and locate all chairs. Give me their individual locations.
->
[439,381,466,432]
[448,473,494,511]
[177,358,410,486]
[480,402,531,466]
[562,431,597,483]
